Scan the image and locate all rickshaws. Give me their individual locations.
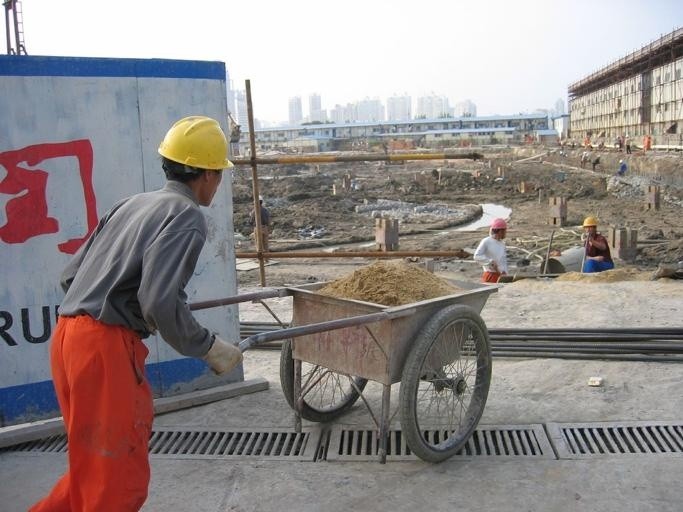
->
[189,275,499,463]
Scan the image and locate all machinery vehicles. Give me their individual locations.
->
[228,112,242,143]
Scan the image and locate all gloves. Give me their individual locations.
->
[198,334,244,377]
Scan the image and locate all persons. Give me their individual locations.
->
[472,217,510,284]
[580,214,614,273]
[23,115,246,512]
[247,194,270,263]
[522,133,651,178]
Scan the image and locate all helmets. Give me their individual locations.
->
[582,217,598,228]
[490,218,507,230]
[156,115,235,171]
